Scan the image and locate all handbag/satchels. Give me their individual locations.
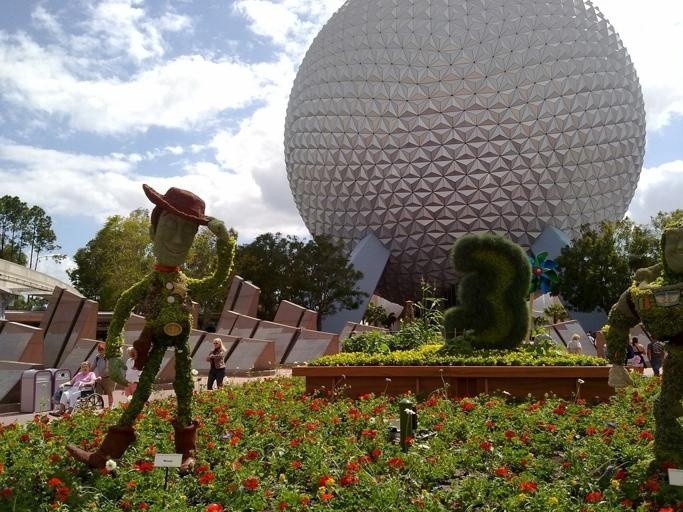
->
[640,356,647,368]
[627,345,633,358]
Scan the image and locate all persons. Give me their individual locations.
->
[123,347,144,403]
[89,340,118,409]
[586,333,595,345]
[566,332,582,356]
[647,334,665,376]
[631,336,646,375]
[65,181,237,480]
[49,360,96,420]
[593,331,607,358]
[205,338,227,390]
[599,217,682,475]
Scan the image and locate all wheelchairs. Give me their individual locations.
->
[54,378,105,417]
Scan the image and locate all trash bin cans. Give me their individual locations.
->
[20,368,72,413]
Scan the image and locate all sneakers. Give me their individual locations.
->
[53,411,64,415]
